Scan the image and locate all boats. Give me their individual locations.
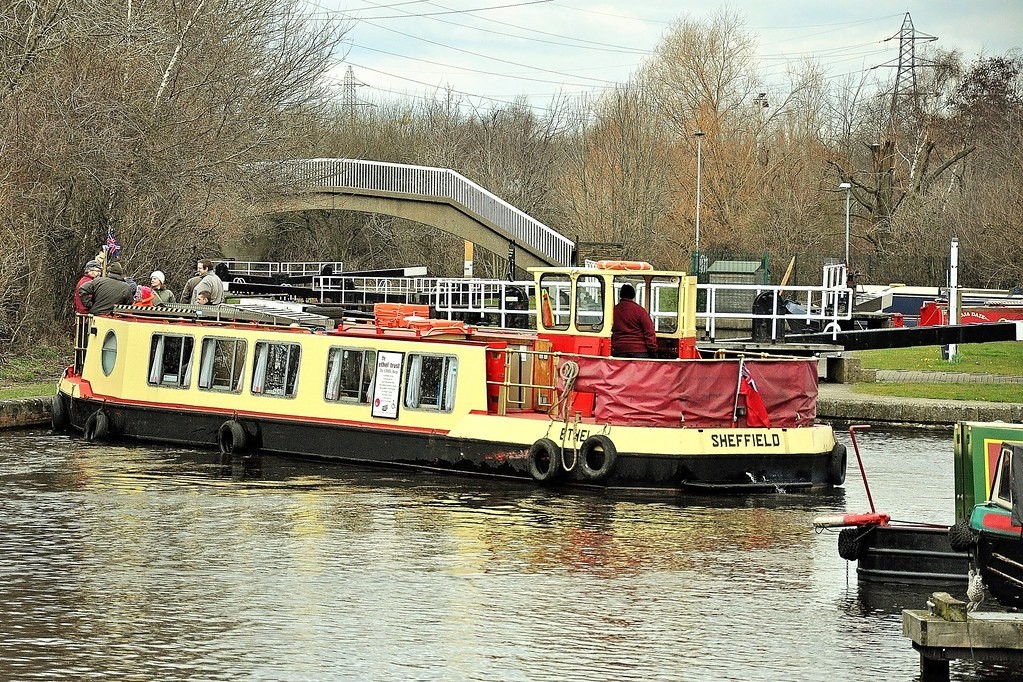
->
[47,237,851,501]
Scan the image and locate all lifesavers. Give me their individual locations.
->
[597,260,653,271]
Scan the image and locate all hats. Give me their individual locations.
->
[85,261,103,270]
[150,271,166,285]
[106,262,122,275]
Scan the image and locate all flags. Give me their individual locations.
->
[105,227,120,262]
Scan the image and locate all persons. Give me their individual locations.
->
[132,284,154,306]
[122,269,137,294]
[192,260,225,304]
[149,270,176,303]
[198,291,213,306]
[180,273,203,303]
[609,283,660,359]
[75,260,103,313]
[78,263,134,316]
